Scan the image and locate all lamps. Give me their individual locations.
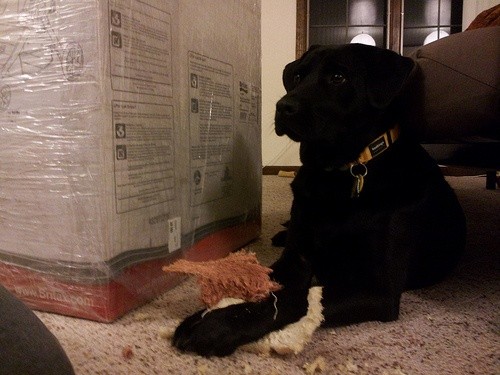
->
[350,32,378,47]
[423,30,450,46]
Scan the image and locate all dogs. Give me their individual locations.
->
[172,43,469,358]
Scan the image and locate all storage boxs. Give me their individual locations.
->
[2,0,264,323]
